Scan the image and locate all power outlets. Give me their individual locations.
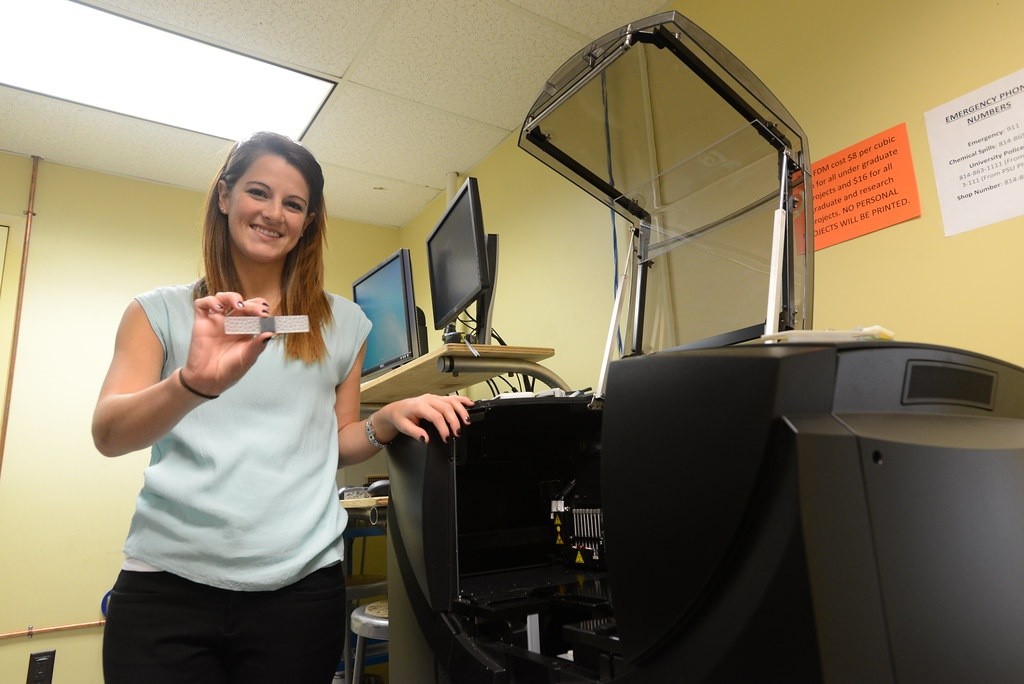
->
[26,650,55,684]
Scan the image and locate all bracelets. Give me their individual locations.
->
[365,415,394,449]
[179,368,222,401]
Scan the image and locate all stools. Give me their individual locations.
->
[349,598,389,684]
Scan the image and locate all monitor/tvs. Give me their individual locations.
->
[425,177,490,330]
[352,248,419,378]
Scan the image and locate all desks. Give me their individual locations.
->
[335,495,389,684]
[359,343,574,422]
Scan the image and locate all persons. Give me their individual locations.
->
[90,131,474,684]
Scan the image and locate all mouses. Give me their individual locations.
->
[367,480,391,497]
